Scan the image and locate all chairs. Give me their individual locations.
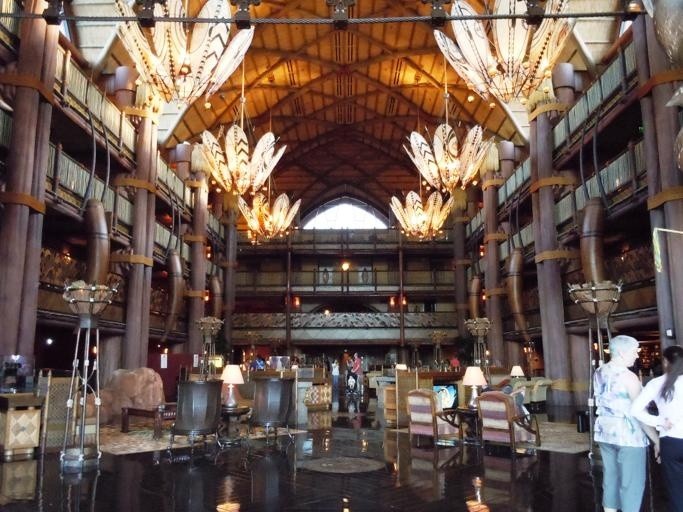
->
[404,389,543,470]
[164,377,225,455]
[242,378,294,446]
[501,375,553,412]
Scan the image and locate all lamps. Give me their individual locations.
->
[220,363,246,409]
[461,364,489,409]
[509,364,526,380]
[212,472,246,512]
[463,474,491,512]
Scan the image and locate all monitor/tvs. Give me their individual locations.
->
[433,383,458,410]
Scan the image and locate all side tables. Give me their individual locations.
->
[220,405,250,442]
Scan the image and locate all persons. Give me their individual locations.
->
[331,357,341,390]
[346,357,357,391]
[290,356,299,369]
[351,351,364,392]
[500,384,531,426]
[592,335,662,512]
[630,346,683,512]
[250,352,267,371]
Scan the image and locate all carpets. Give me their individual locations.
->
[513,421,597,454]
[96,422,306,457]
